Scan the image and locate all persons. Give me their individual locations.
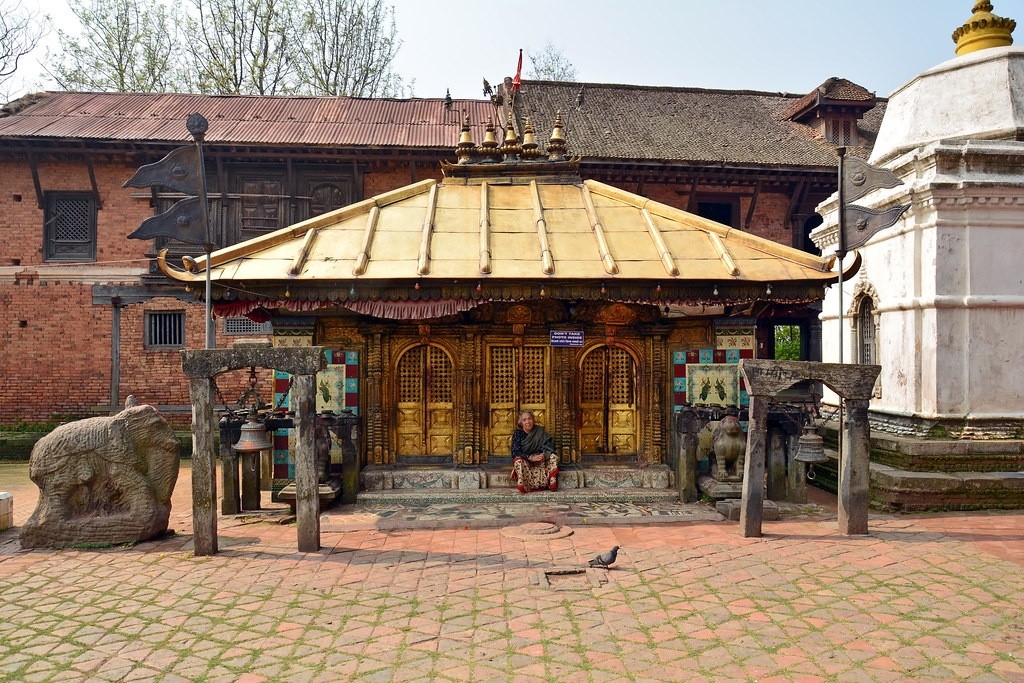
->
[509,410,559,493]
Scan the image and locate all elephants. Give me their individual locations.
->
[27,397,180,523]
